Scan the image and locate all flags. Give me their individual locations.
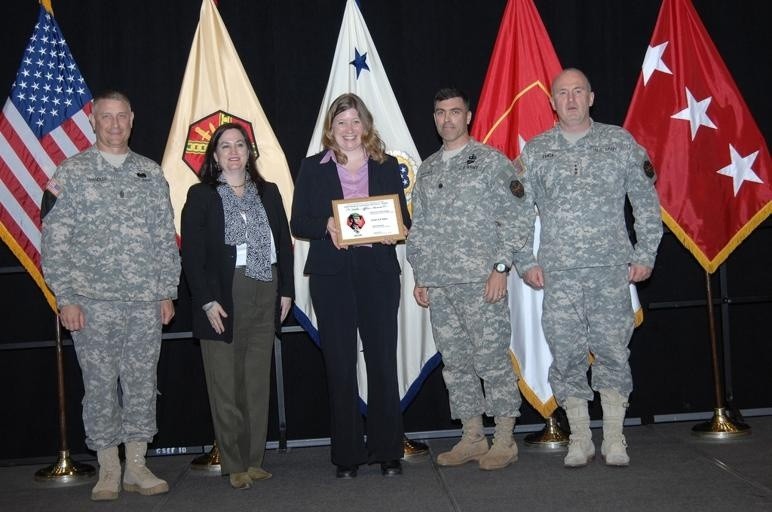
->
[1,10,97,314]
[622,0,772,274]
[161,2,293,248]
[471,0,645,419]
[293,0,441,416]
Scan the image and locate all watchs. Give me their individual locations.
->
[493,262,509,273]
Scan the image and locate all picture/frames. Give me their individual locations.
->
[331,193,405,245]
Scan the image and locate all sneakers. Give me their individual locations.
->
[229,471,254,489]
[248,467,272,481]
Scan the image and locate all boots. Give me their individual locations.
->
[90,446,122,500]
[123,440,170,497]
[436,416,489,466]
[563,397,596,468]
[478,417,519,469]
[599,393,631,466]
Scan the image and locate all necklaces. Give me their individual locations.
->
[227,182,246,191]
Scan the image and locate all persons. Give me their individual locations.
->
[289,93,412,478]
[514,69,664,465]
[177,123,295,490]
[40,91,182,503]
[405,89,522,472]
[347,212,363,231]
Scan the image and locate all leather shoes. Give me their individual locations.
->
[381,460,403,477]
[336,463,358,479]
